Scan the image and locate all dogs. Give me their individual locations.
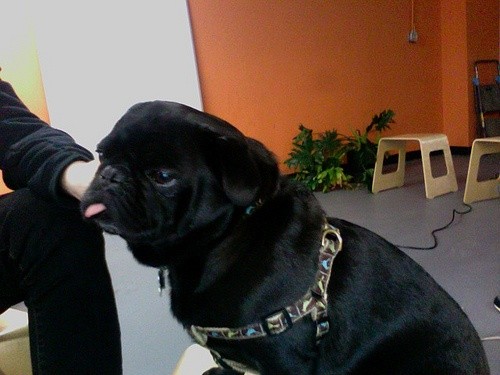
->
[80,101,492,375]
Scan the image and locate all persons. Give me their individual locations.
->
[0,76,127,375]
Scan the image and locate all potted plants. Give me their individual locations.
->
[285,101,398,194]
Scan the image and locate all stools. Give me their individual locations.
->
[463,137,500,204]
[372,134,459,200]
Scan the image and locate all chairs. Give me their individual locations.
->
[474,58,500,138]
